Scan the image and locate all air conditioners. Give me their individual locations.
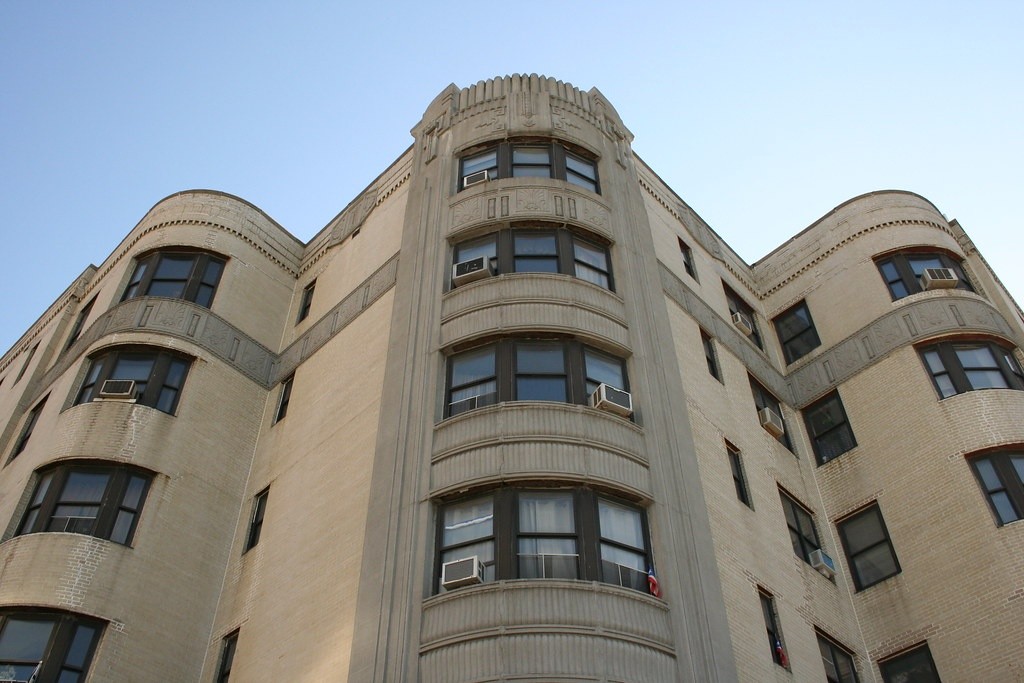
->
[100,379,139,401]
[464,169,491,189]
[758,407,785,438]
[442,555,484,590]
[591,383,633,417]
[452,255,494,287]
[731,312,752,336]
[919,267,959,291]
[808,549,837,579]
